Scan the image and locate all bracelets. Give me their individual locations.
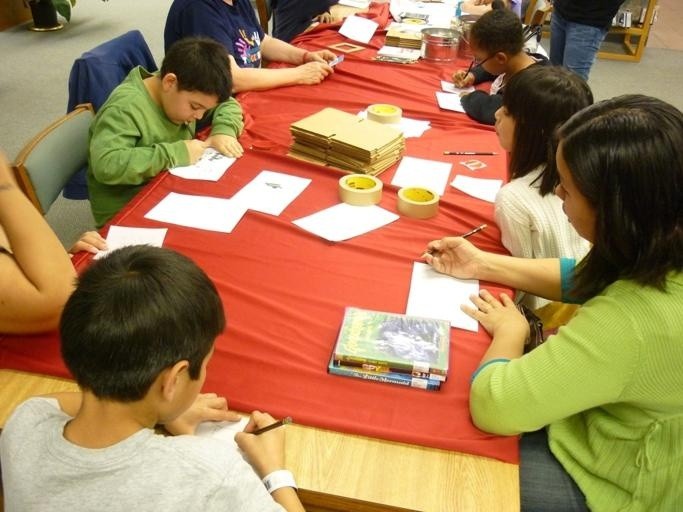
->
[302,51,308,63]
[264,468,297,495]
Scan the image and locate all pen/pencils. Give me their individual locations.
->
[254,417,292,435]
[443,151,499,155]
[418,224,488,259]
[459,61,474,88]
[184,121,196,139]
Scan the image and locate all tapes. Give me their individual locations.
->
[367,103,402,124]
[397,186,440,219]
[339,174,383,206]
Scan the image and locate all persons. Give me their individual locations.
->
[494,62,596,352]
[0,152,106,334]
[422,93,683,512]
[86,36,245,227]
[462,0,522,20]
[268,0,340,43]
[451,7,550,123]
[0,244,307,512]
[164,0,337,94]
[549,0,624,82]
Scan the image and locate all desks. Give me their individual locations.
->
[1,0,521,512]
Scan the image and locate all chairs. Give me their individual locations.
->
[524,0,554,32]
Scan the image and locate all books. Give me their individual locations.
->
[385,21,424,49]
[327,308,451,390]
[287,107,405,179]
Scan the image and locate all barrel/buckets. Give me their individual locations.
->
[421,28,464,62]
[458,15,483,61]
